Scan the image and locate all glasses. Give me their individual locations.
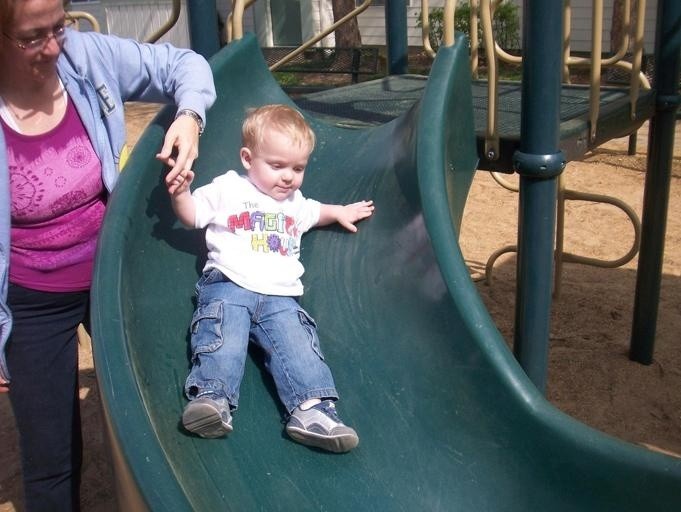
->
[4,10,76,51]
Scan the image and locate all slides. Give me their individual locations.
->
[90,31,680,512]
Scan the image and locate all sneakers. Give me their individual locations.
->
[286,399,358,454]
[181,397,235,440]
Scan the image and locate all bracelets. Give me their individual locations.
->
[173,109,205,139]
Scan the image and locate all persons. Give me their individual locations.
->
[156,102,375,454]
[2,1,218,512]
